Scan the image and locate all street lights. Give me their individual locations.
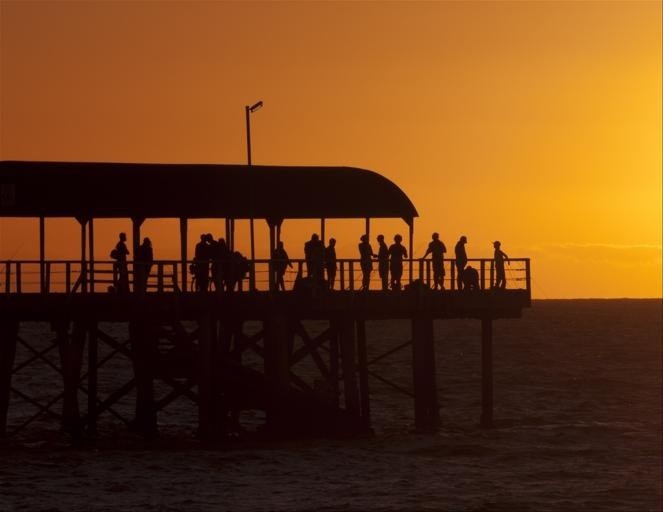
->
[242,98,265,285]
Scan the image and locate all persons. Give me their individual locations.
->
[140,237,153,291]
[192,233,509,292]
[115,232,131,293]
